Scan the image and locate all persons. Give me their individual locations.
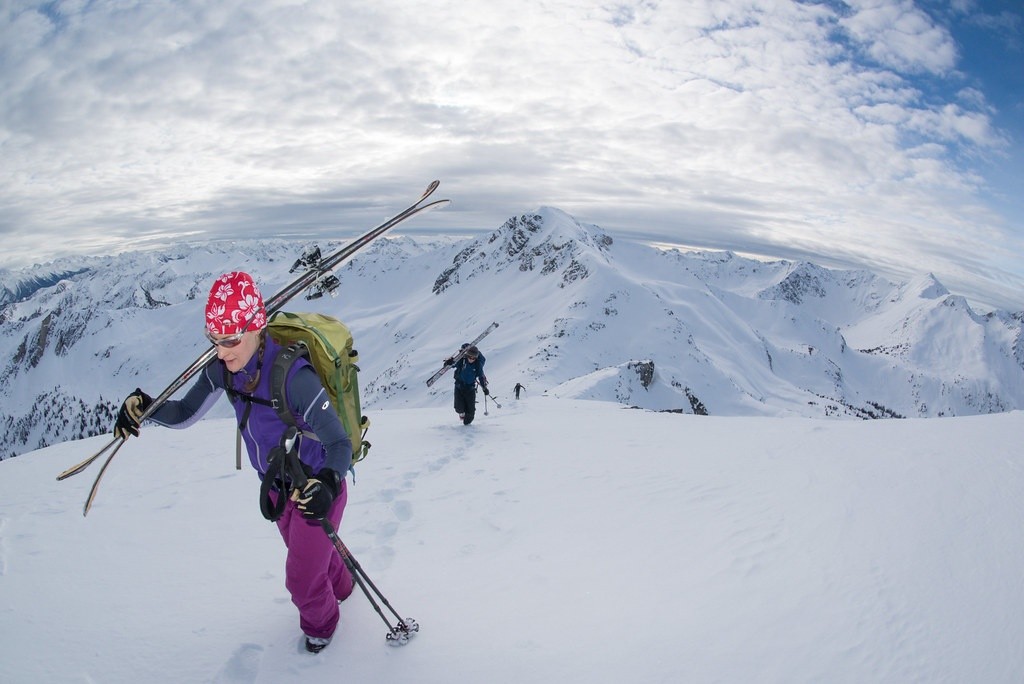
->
[513,383,525,400]
[444,344,488,425]
[113,272,357,652]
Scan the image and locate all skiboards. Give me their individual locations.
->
[426,322,500,387]
[55,180,452,518]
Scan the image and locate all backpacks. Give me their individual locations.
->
[265,310,372,486]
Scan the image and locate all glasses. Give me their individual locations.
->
[204,307,262,348]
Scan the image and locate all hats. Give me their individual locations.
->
[204,272,268,334]
[466,345,479,358]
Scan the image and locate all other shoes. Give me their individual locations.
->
[459,412,465,418]
[305,636,332,653]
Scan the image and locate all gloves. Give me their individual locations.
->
[113,388,152,441]
[446,358,453,365]
[289,467,338,520]
[483,389,489,395]
